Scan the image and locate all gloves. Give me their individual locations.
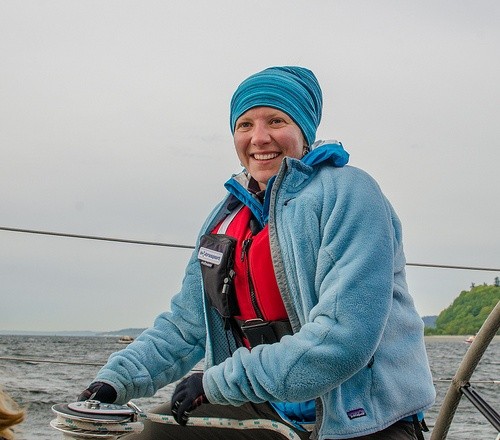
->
[77,382,117,404]
[170,373,210,426]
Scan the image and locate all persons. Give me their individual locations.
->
[78,66,437,440]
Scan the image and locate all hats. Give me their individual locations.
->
[229,66,324,149]
